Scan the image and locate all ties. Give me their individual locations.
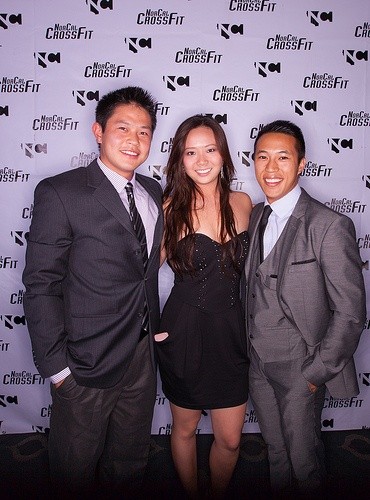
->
[259,205,273,267]
[125,183,149,332]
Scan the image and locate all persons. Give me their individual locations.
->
[157,114,254,500]
[240,120,367,500]
[21,86,168,500]
